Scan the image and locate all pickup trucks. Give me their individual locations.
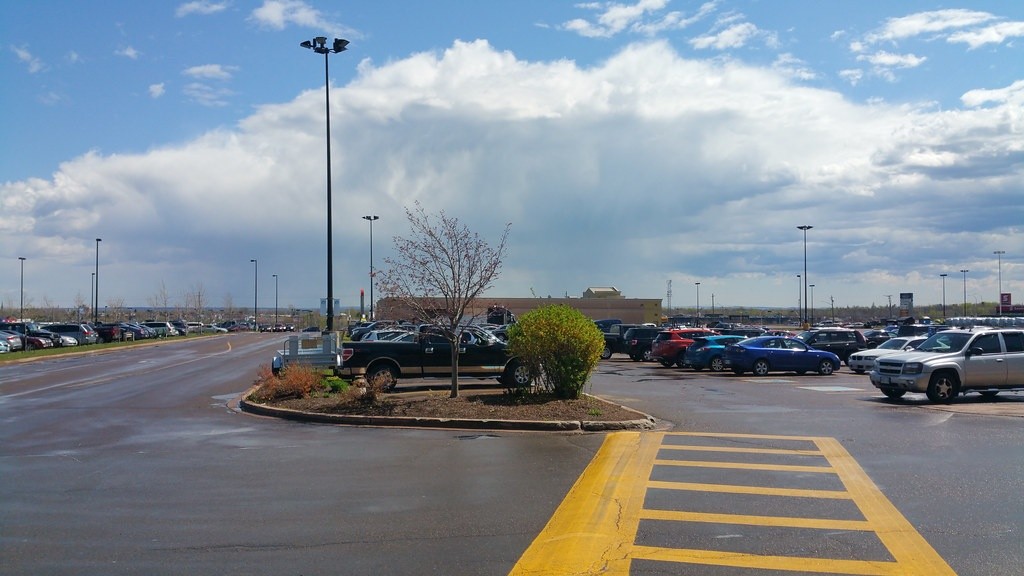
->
[337,323,535,393]
[599,323,648,360]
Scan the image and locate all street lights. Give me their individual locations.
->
[251,259,278,328]
[695,283,700,328]
[796,225,815,326]
[18,258,26,320]
[301,37,380,331]
[940,270,969,318]
[91,239,101,324]
[994,251,1005,315]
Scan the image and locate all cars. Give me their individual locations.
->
[685,324,952,376]
[349,320,417,341]
[643,323,687,329]
[0,318,320,355]
[719,323,797,337]
[480,323,509,343]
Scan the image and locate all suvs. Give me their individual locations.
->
[623,328,665,362]
[592,319,622,333]
[870,325,1024,405]
[651,328,721,368]
